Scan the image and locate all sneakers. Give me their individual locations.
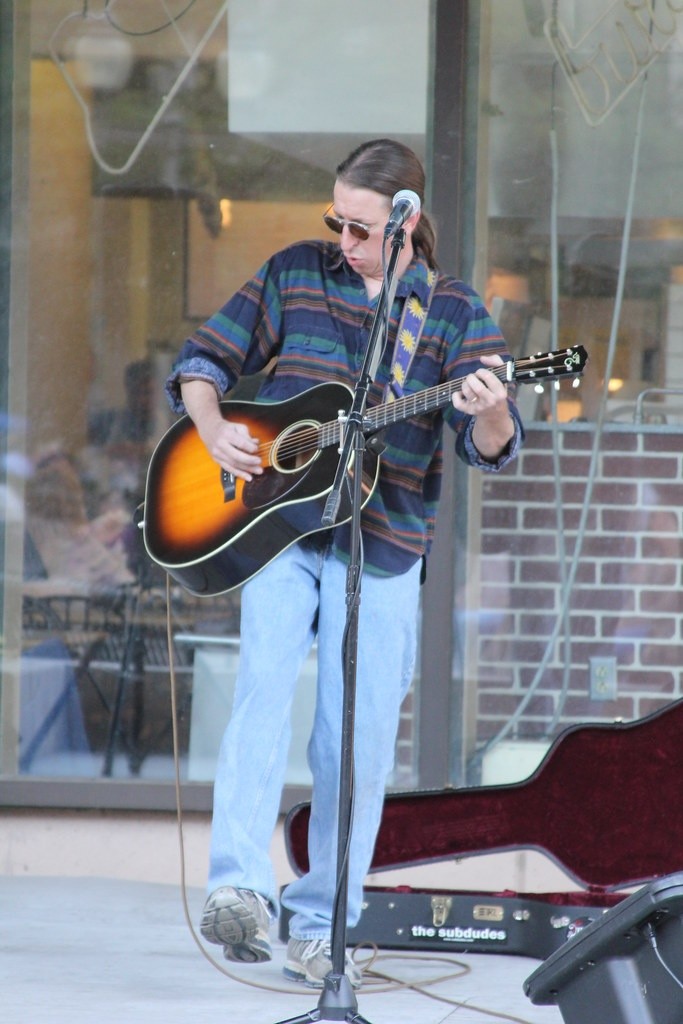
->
[200,886,273,964]
[284,938,363,989]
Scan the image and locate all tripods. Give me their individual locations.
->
[19,560,190,780]
[277,227,405,1024]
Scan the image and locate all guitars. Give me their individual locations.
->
[143,343,589,597]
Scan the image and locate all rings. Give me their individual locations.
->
[471,396,477,403]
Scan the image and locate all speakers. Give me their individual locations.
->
[521,869,683,1024]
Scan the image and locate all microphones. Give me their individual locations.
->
[384,189,421,240]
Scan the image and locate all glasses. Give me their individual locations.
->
[322,203,371,240]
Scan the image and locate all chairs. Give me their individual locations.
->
[19,552,148,778]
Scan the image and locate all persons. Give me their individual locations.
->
[162,138,526,989]
[86,358,158,461]
